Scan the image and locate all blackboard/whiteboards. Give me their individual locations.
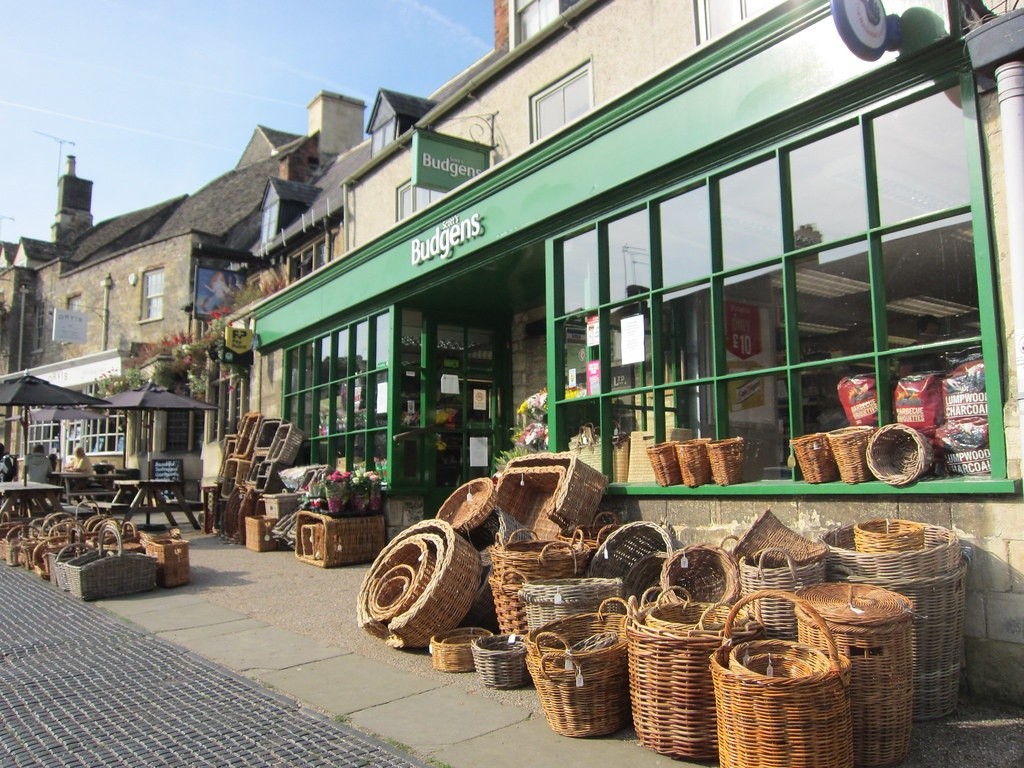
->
[151,458,184,504]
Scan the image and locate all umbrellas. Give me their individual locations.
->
[5,404,108,472]
[82,378,222,524]
[0,368,112,488]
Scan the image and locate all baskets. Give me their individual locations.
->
[436,477,497,532]
[430,422,966,768]
[0,500,157,601]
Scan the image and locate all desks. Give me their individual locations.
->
[0,472,204,531]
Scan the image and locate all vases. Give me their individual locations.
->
[324,487,381,512]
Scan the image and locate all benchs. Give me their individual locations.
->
[165,496,204,511]
[68,490,132,496]
[60,501,94,516]
[88,500,129,511]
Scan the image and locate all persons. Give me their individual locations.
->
[27,443,57,484]
[0,443,14,481]
[900,315,941,378]
[67,446,93,503]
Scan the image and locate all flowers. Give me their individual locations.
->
[323,471,381,487]
[493,387,548,471]
